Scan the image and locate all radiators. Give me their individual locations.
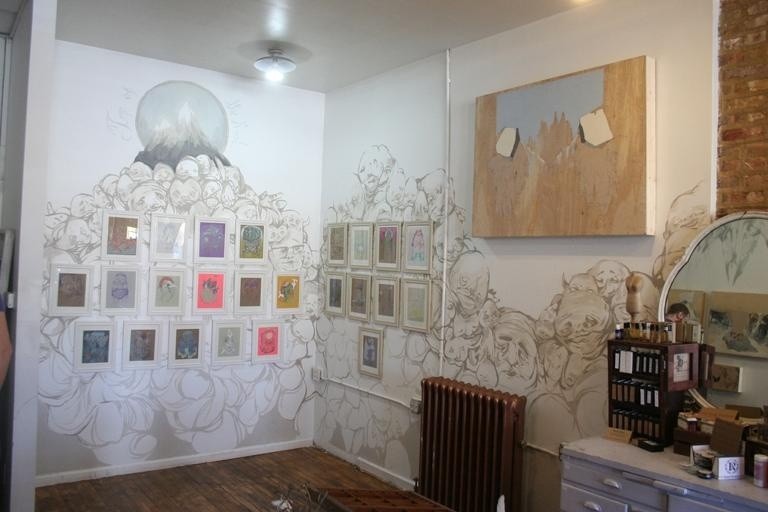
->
[405,376,529,512]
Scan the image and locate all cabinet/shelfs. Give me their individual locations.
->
[557,435,768,512]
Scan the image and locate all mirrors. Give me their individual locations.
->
[657,211,768,429]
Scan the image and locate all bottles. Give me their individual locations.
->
[754,454,767,487]
